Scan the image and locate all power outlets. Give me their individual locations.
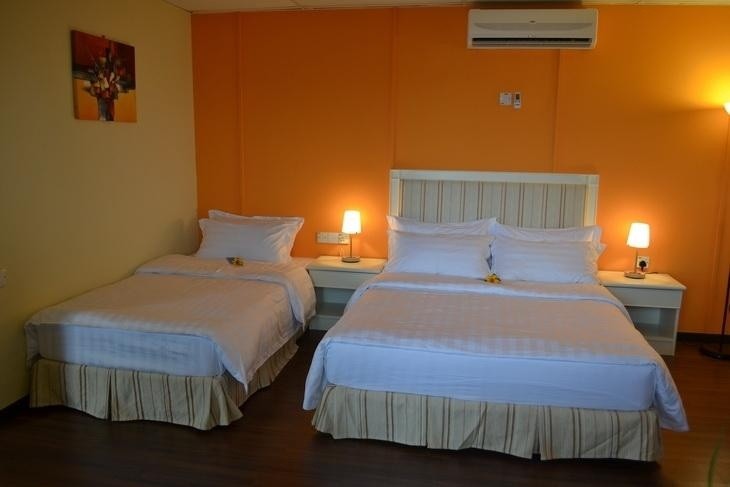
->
[637,257,650,269]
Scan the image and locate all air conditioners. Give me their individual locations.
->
[466,8,598,49]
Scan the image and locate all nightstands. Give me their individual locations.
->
[308,255,389,332]
[597,269,687,357]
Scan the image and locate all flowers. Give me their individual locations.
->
[82,42,131,118]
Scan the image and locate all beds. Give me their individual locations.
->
[303,168,692,462]
[22,256,316,430]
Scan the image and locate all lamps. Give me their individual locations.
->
[698,101,730,360]
[626,222,650,279]
[341,210,362,263]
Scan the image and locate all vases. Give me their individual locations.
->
[96,96,115,121]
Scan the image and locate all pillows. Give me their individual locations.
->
[491,239,606,282]
[384,213,498,236]
[494,224,604,243]
[385,230,493,282]
[196,209,304,263]
[208,210,251,223]
